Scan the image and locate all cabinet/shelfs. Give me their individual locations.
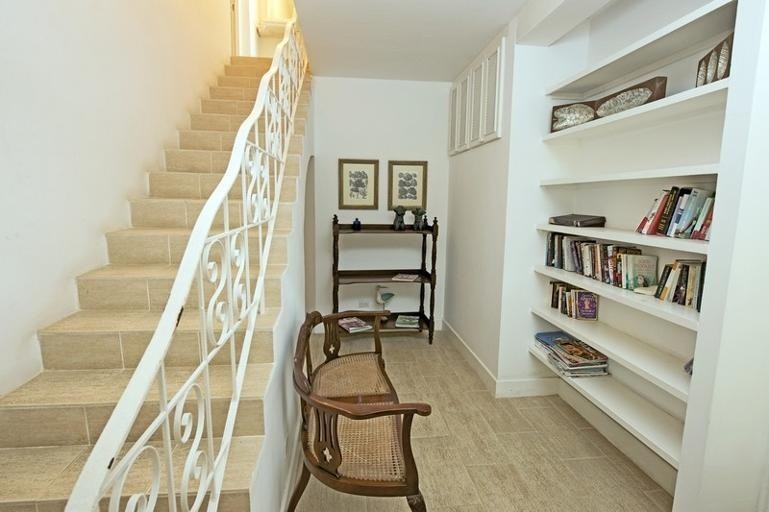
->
[332,214,439,345]
[528,0,767,499]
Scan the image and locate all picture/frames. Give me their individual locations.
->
[337,158,428,212]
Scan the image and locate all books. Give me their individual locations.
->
[339,316,373,333]
[391,273,418,282]
[395,314,420,328]
[532,186,713,381]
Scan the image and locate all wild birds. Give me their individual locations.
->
[375,285,395,317]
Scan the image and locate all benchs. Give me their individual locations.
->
[286,309,432,511]
